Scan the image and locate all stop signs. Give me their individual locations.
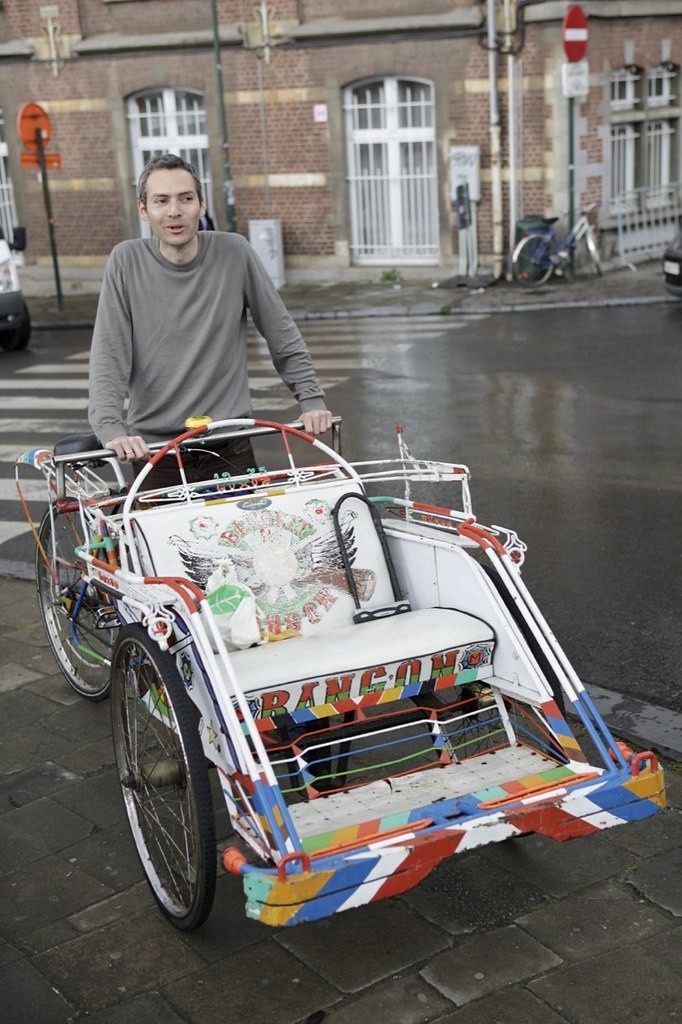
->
[562,5,589,63]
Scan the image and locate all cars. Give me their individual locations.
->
[0,223,33,351]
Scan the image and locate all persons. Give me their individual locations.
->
[81,152,337,503]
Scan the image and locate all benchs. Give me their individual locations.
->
[124,419,497,730]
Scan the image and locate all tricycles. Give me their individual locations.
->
[13,416,669,933]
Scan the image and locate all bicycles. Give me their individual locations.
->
[512,201,606,293]
